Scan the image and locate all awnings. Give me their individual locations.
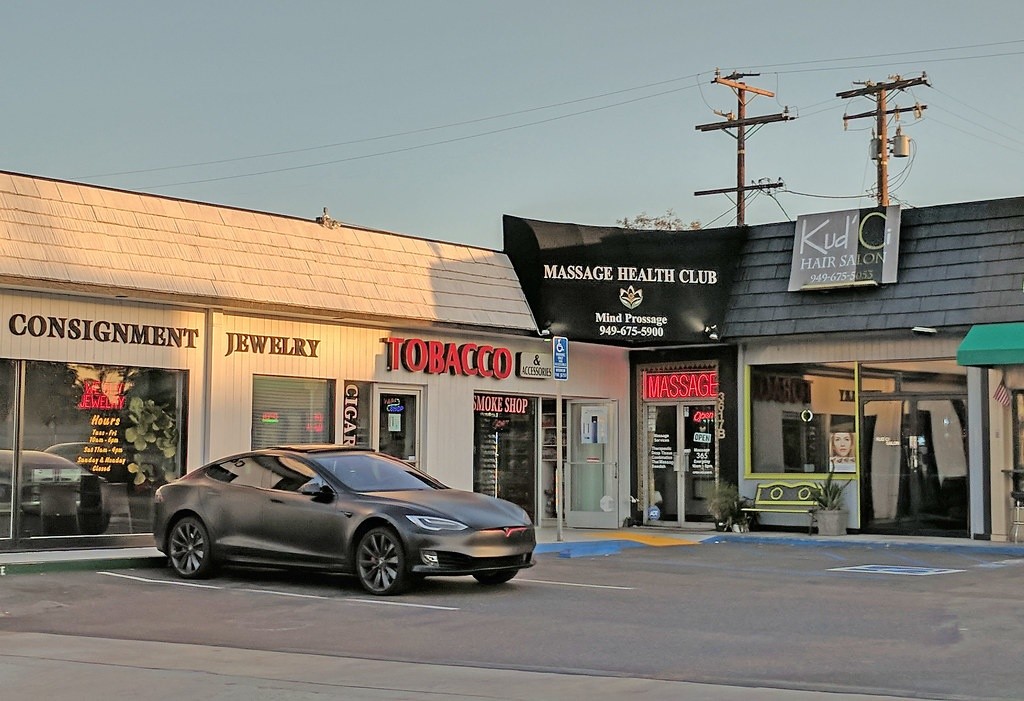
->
[956,322,1024,365]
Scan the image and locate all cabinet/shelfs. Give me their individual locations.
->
[496,427,533,521]
[542,413,567,462]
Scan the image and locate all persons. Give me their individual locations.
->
[831,433,853,457]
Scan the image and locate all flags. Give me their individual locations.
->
[993,379,1010,407]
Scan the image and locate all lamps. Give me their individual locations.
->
[704,324,723,340]
[912,326,938,337]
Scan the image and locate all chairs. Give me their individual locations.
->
[23,481,80,536]
[99,482,137,533]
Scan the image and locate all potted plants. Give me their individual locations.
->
[813,459,849,536]
[701,479,736,531]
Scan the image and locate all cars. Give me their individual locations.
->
[43,442,135,481]
[0,449,111,538]
[153,443,537,596]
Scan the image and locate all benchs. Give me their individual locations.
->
[738,481,824,536]
